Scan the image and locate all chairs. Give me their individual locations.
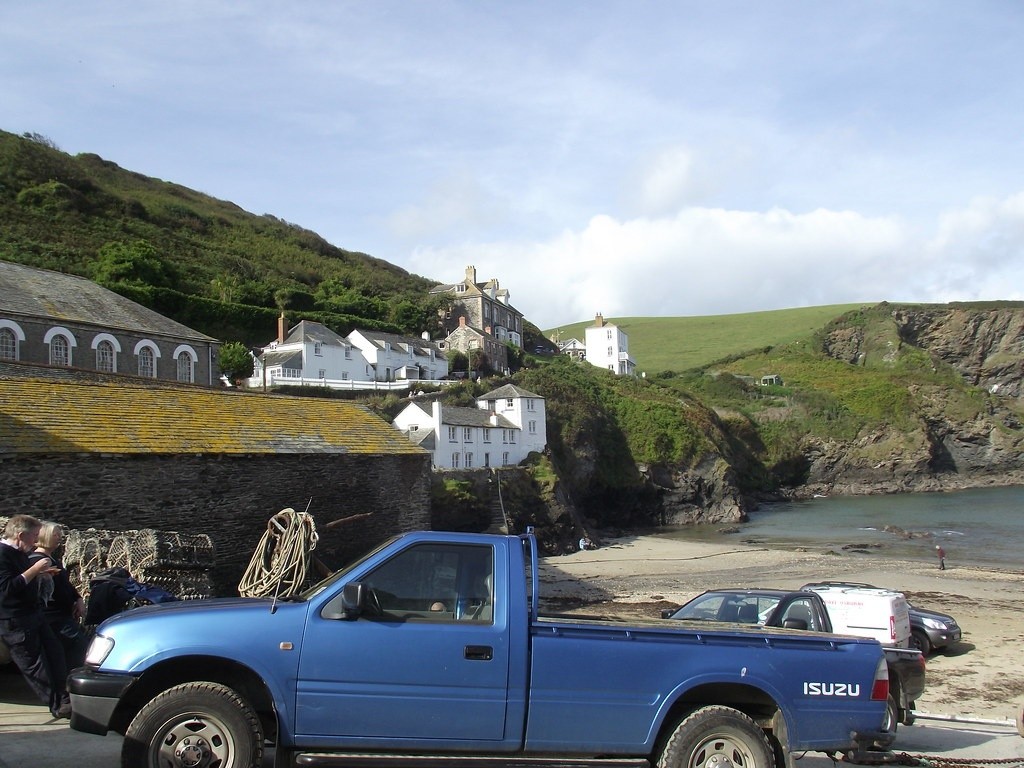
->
[463,568,493,620]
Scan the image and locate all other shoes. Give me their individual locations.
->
[55,703,72,719]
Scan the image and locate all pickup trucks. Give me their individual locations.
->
[657,588,926,750]
[66,529,899,768]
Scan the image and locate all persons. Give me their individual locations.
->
[579,537,588,550]
[935,545,947,571]
[0,514,68,716]
[27,523,88,666]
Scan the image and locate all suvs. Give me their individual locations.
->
[799,580,962,659]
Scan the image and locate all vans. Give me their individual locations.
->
[756,587,911,650]
